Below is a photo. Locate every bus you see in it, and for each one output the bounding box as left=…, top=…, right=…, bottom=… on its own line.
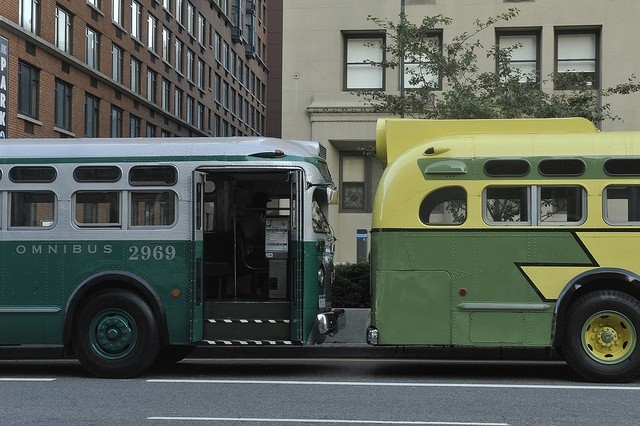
left=0, top=137, right=347, bottom=379
left=366, top=117, right=639, bottom=384
